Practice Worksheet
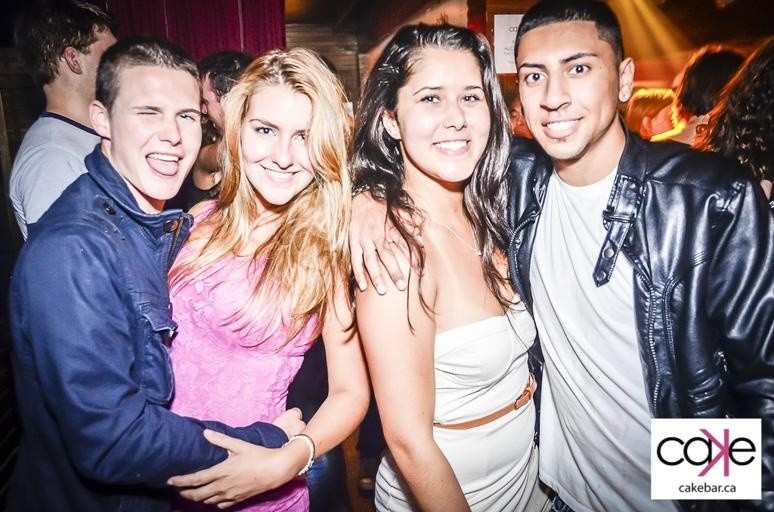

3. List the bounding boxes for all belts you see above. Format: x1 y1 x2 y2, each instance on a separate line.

434 374 537 429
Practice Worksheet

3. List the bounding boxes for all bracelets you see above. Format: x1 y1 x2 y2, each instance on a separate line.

285 433 316 476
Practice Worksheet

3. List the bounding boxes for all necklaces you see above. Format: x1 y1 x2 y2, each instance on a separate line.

438 218 482 259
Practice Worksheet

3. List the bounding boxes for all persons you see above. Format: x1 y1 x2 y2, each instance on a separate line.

160 46 371 510
348 0 774 510
354 20 558 512
9 0 774 238
8 38 308 511
